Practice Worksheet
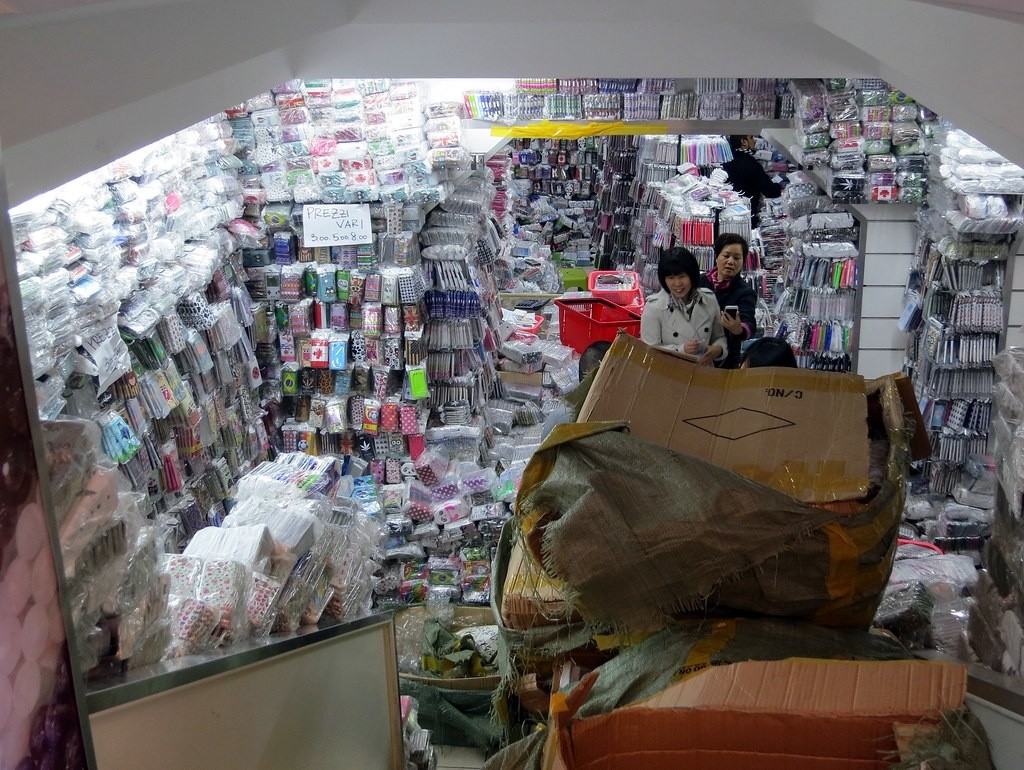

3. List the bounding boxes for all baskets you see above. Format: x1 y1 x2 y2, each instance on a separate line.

500 311 544 341
588 271 645 315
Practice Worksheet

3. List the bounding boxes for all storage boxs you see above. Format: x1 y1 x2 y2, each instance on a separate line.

498 372 543 385
501 332 967 770
395 606 502 690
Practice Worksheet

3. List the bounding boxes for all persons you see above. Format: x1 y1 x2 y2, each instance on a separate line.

723 135 781 230
640 247 728 367
699 233 757 369
540 340 613 444
739 337 798 369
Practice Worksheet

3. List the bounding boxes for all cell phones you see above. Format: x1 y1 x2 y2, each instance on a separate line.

723 306 738 320
